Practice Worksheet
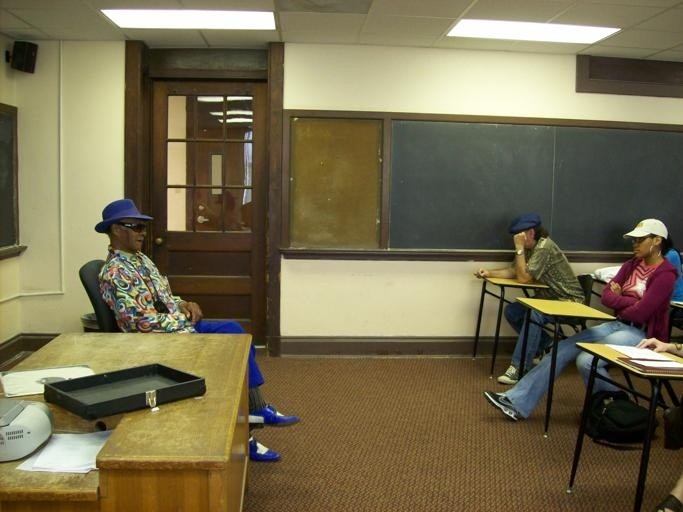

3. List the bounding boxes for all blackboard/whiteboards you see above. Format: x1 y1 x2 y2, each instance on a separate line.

1 104 19 258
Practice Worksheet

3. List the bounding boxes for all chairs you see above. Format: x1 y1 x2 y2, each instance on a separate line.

79 260 120 332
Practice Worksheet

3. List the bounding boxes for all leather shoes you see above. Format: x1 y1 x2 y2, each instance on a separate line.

249 404 299 429
249 436 280 461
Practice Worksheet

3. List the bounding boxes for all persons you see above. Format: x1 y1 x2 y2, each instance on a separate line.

482 217 679 423
634 337 683 358
471 212 586 385
660 234 683 341
650 473 683 512
93 199 302 463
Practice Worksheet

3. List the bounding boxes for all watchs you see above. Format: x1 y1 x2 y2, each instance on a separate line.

513 250 524 256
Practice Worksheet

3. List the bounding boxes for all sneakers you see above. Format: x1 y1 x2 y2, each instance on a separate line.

484 392 519 421
498 342 555 384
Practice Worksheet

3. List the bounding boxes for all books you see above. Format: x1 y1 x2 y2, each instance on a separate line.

615 356 683 375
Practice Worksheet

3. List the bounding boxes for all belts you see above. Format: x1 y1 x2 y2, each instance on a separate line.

618 319 648 332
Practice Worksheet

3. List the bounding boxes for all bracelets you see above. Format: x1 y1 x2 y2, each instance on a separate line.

674 343 682 354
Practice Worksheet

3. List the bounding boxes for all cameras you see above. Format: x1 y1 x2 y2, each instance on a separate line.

153 300 171 314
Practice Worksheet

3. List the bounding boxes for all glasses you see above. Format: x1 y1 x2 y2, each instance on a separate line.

118 222 144 232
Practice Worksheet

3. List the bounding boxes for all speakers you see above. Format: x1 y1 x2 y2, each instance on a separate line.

12 40 39 73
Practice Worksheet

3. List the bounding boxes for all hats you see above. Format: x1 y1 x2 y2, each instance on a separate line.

509 213 540 232
623 218 668 240
95 199 154 232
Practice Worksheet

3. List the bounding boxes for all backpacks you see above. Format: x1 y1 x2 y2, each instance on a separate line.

584 391 658 450
